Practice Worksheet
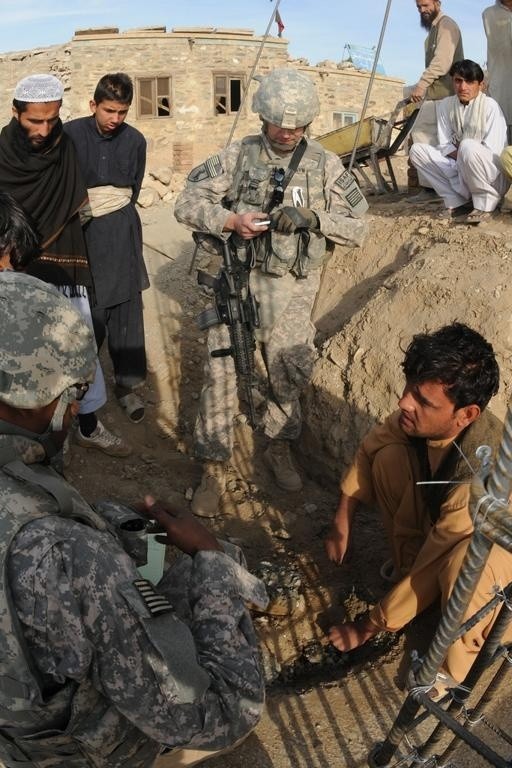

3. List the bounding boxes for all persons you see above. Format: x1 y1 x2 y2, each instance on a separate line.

409 60 510 224
501 146 512 213
172 70 369 519
0 189 44 271
0 73 133 457
63 74 150 424
1 273 271 768
481 0 511 130
408 0 464 107
327 316 512 707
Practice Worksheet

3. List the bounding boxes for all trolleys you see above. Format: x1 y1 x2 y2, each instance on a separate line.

310 95 419 197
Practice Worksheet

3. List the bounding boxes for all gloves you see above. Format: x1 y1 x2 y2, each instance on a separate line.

269 206 318 233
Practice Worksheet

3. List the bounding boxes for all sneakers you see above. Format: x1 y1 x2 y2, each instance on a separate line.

76 420 132 458
404 186 443 204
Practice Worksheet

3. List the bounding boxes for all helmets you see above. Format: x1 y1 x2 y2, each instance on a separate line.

0 271 97 410
251 69 320 130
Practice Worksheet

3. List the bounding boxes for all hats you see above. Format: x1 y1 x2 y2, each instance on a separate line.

14 74 65 103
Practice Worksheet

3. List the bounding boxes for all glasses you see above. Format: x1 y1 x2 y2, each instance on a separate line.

74 382 89 400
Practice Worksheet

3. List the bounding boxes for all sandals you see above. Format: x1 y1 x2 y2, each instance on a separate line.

437 204 472 219
465 207 492 223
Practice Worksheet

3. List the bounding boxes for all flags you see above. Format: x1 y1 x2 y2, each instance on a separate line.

275 9 284 37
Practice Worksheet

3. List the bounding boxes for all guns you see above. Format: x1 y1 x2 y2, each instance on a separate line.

197 241 259 432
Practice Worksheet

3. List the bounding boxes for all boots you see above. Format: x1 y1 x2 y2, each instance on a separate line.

263 440 304 492
190 460 226 518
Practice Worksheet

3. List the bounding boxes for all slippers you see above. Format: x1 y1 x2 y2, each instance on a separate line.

118 392 145 424
